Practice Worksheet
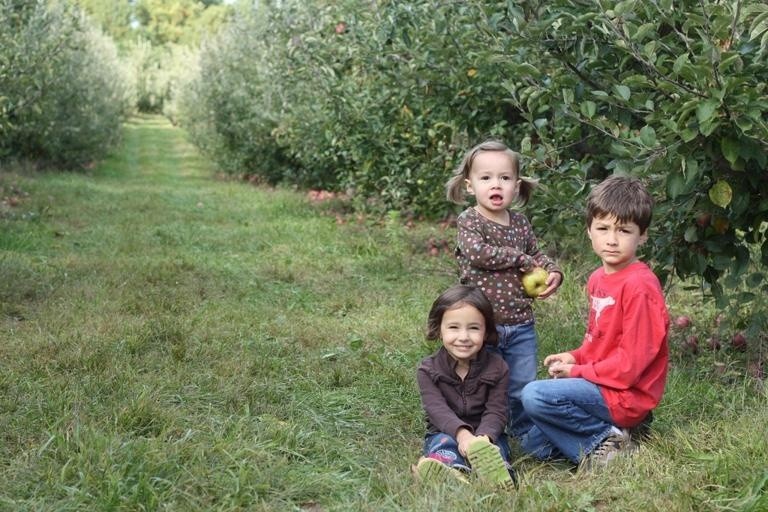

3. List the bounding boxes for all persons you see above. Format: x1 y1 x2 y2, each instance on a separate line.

412 284 520 498
514 174 671 475
441 140 566 440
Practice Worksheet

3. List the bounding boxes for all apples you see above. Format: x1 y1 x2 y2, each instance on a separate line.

714 313 723 327
337 23 346 32
679 334 698 355
218 165 455 256
521 266 550 298
733 334 747 352
676 316 690 327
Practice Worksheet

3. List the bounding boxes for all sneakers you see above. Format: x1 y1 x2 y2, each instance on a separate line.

465 438 516 495
588 427 626 468
414 456 471 495
